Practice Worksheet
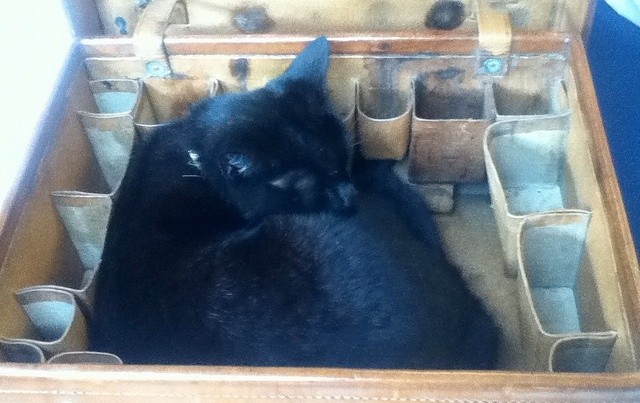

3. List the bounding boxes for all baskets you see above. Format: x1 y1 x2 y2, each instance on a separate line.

0 0 640 403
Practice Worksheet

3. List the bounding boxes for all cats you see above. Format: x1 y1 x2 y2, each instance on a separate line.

86 35 502 369
